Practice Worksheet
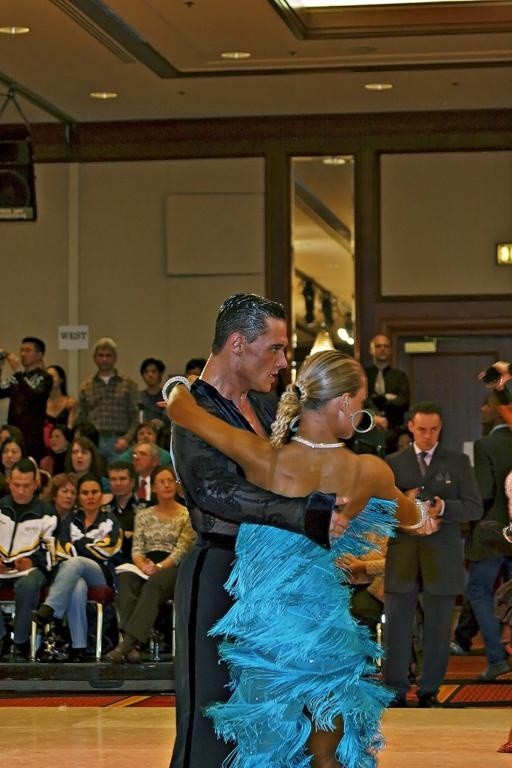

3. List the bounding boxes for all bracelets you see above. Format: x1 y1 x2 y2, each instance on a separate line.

398 497 425 530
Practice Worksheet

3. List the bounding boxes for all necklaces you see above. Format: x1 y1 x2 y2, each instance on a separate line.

291 435 346 449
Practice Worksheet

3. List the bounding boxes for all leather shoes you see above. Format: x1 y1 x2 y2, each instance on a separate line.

417 693 447 708
387 696 407 708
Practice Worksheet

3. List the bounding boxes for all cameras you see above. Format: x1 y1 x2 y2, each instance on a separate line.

482 367 501 384
416 491 435 506
0 351 9 360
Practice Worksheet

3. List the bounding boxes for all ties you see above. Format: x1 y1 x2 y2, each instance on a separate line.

373 371 386 417
138 478 149 500
418 451 430 479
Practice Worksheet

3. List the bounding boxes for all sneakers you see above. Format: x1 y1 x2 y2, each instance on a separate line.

13 642 31 663
60 647 88 663
101 642 128 663
449 641 472 655
130 650 142 661
478 662 511 681
29 604 53 625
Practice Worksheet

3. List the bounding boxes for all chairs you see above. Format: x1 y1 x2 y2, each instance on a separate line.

0 580 175 661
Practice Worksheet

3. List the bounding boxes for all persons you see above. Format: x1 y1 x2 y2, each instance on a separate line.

453 393 510 681
364 335 409 453
377 401 484 708
1 337 208 663
161 351 429 768
478 360 511 418
170 293 349 768
